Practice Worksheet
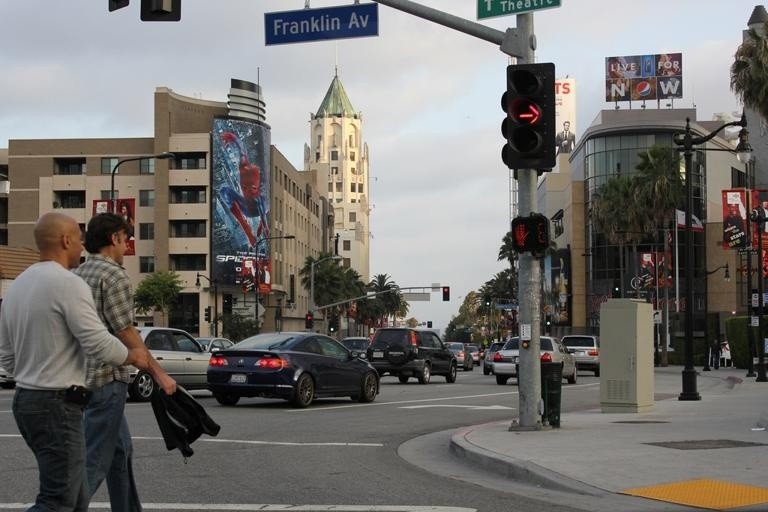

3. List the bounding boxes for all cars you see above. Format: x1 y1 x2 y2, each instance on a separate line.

204 331 380 408
481 334 600 386
127 326 235 403
339 328 480 384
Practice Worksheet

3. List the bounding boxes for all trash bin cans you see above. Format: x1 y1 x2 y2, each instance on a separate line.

515 360 560 427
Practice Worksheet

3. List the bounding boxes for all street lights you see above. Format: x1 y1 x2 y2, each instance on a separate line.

111 152 175 214
255 235 295 334
310 256 342 332
195 272 218 337
673 109 753 401
703 262 730 371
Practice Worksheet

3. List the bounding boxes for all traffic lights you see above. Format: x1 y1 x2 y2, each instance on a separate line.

443 287 450 301
305 313 314 329
328 321 337 333
499 63 556 168
510 213 551 255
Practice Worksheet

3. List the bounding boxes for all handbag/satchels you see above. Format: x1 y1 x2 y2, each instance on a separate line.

65 385 93 411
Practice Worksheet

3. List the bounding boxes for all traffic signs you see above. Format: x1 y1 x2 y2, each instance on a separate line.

476 0 562 21
264 3 380 46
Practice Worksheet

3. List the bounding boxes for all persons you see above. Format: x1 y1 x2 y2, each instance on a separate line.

118 203 135 227
0 212 152 512
67 213 177 512
754 192 768 235
